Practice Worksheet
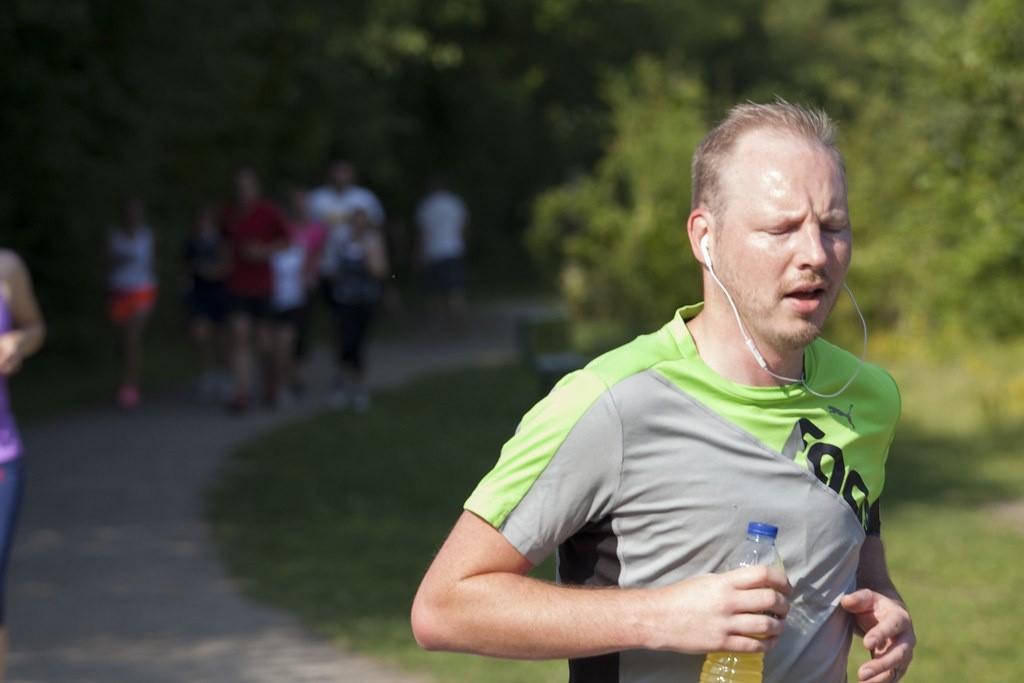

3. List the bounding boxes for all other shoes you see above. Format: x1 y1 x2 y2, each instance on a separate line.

118 386 141 408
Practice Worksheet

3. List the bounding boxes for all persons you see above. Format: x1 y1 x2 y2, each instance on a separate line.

410 101 917 683
204 155 390 414
414 173 474 327
0 248 45 683
107 196 158 412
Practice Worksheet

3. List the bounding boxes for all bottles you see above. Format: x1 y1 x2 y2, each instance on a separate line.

699 523 786 683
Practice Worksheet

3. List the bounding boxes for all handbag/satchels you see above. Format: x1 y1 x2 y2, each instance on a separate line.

333 268 378 303
